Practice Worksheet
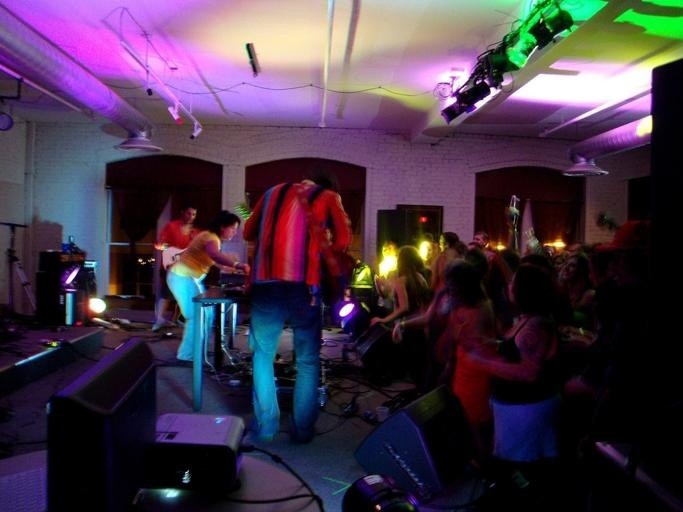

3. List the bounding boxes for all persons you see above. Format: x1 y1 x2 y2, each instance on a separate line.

371 210 646 468
149 205 204 331
165 209 251 364
242 173 353 443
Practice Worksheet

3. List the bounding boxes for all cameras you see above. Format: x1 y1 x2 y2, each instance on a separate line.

342 474 418 511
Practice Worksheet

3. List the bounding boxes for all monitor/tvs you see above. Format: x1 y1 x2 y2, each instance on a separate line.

45 337 154 511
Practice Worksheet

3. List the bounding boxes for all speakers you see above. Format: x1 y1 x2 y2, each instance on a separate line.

353 322 407 371
376 209 405 243
355 382 475 505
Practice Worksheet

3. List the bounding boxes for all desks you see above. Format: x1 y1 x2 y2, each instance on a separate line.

0 449 323 512
185 291 330 415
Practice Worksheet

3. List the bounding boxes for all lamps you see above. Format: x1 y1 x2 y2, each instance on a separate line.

111 129 163 154
120 40 206 141
87 292 108 329
563 154 610 180
334 297 361 323
439 0 573 127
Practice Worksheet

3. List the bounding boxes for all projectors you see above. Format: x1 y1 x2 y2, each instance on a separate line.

151 412 246 494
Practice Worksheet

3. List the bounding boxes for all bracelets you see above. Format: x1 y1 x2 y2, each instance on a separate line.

231 261 240 270
